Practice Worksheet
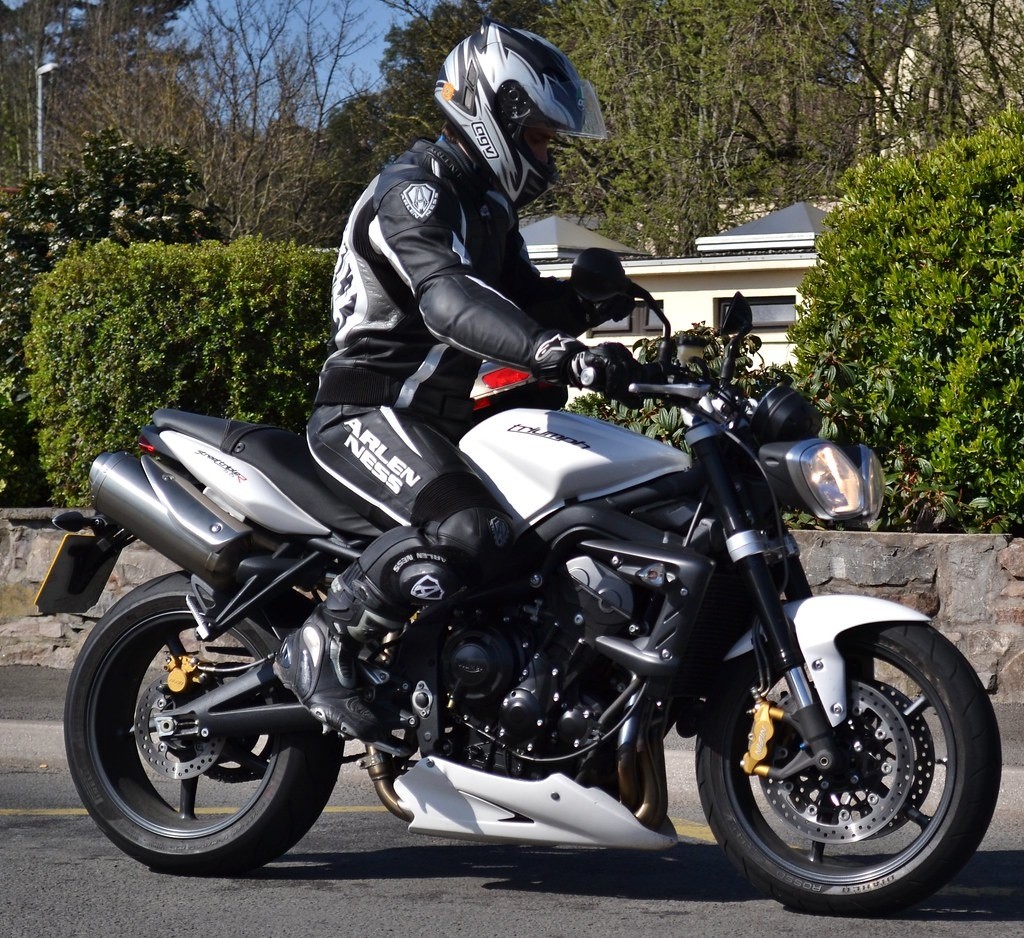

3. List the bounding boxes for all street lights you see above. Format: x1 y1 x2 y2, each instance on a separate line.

35 61 60 174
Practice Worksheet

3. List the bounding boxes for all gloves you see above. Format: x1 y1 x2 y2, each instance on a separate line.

568 346 647 408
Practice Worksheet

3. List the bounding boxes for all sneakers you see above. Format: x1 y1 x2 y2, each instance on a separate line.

274 611 378 745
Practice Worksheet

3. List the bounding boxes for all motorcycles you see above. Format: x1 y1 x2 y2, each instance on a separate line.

31 243 1003 912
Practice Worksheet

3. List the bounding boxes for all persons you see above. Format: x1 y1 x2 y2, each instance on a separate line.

270 23 658 744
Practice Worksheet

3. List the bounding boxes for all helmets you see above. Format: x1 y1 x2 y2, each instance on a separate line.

433 17 607 210
